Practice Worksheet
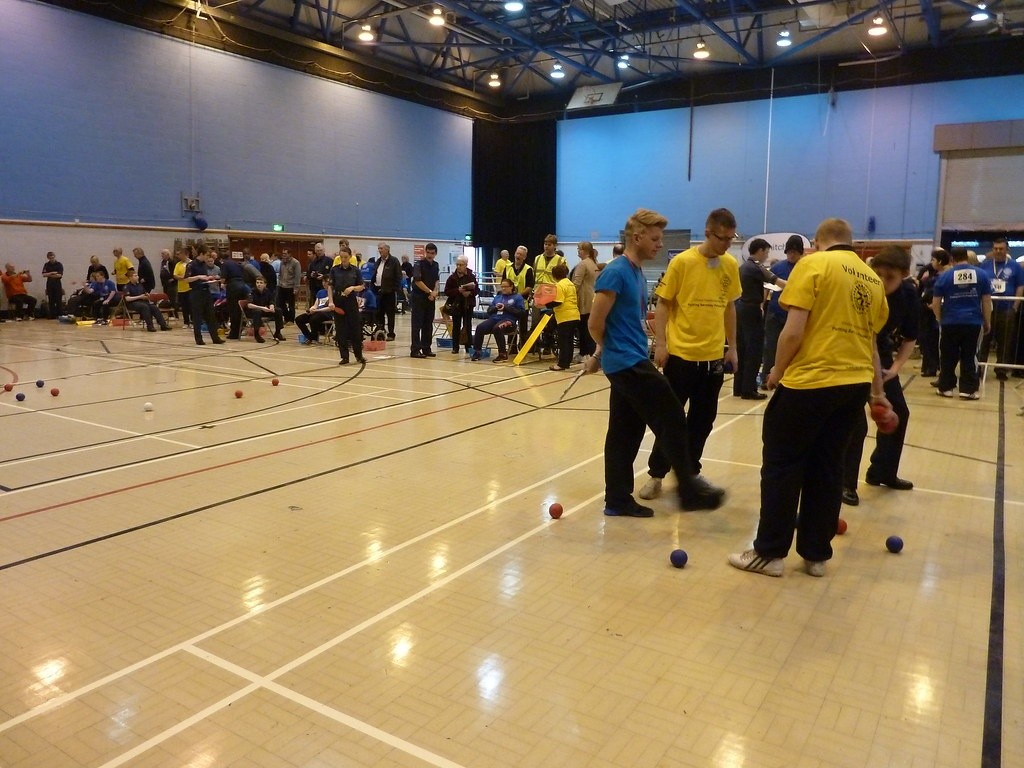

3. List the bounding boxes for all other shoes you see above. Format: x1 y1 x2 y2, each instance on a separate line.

411 352 425 358
690 474 724 496
930 379 940 387
452 350 459 354
492 353 509 363
424 352 436 357
549 365 564 370
15 313 395 365
734 391 767 400
472 353 480 362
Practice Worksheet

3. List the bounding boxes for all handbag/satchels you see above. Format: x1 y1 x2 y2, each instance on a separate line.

443 298 458 316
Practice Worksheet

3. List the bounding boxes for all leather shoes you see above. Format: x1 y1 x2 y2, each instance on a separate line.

864 472 913 490
842 486 859 506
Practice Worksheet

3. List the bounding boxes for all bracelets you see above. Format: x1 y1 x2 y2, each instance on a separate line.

592 353 600 361
428 289 432 294
872 393 887 399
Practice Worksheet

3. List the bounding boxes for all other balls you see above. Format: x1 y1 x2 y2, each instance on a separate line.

670 549 688 568
36 380 44 388
51 388 60 396
272 379 279 385
549 503 563 518
16 393 25 401
837 519 848 535
144 402 153 410
886 536 904 553
877 410 898 434
235 390 243 397
4 383 13 392
871 406 885 421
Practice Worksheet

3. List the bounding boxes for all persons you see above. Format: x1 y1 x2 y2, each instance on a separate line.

240 246 301 343
733 233 804 399
917 238 1024 399
444 255 479 354
58 256 122 326
841 243 920 506
588 208 725 518
355 252 376 322
42 252 64 319
111 247 177 332
728 219 890 576
398 254 413 315
329 239 366 365
303 244 334 302
409 242 440 358
168 244 245 345
295 274 334 345
471 234 607 371
638 207 743 501
370 242 402 341
2 261 38 321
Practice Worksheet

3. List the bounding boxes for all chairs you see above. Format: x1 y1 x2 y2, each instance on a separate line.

482 319 523 360
238 300 277 340
432 313 453 346
306 315 337 347
644 311 656 370
6 288 178 331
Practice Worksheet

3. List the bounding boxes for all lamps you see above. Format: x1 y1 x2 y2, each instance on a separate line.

971 0 989 22
776 24 791 46
693 37 709 59
617 49 631 68
550 60 565 78
358 19 374 41
867 11 888 36
429 4 445 26
488 70 501 87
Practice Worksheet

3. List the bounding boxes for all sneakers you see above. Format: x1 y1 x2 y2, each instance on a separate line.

682 490 721 511
728 550 784 577
604 494 654 518
959 392 979 399
638 477 662 499
803 561 826 577
936 390 953 397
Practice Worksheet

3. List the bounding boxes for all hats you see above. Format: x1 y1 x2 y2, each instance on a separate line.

784 235 804 256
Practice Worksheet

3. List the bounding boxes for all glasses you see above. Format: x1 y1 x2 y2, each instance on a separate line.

501 286 510 288
711 232 739 244
456 263 465 266
